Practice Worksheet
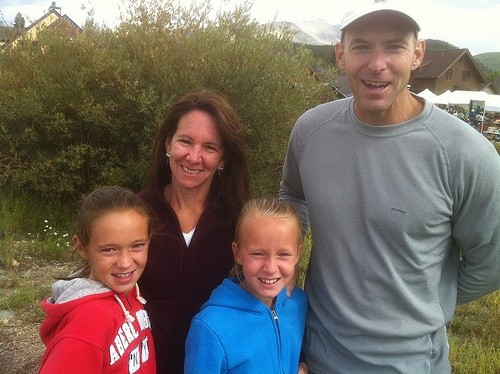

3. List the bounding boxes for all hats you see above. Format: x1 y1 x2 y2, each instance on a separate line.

339 0 420 30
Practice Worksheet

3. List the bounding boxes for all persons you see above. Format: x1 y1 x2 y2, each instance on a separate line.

37 186 160 373
184 196 309 373
134 91 310 374
278 1 500 373
